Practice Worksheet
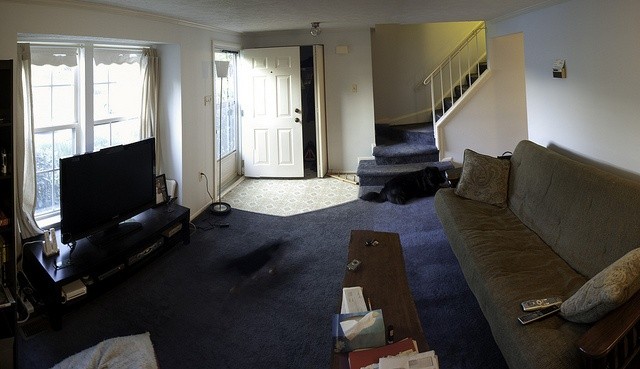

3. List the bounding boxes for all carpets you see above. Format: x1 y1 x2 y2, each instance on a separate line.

13 190 508 369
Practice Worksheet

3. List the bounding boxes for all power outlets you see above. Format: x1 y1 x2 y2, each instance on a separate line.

200 170 205 176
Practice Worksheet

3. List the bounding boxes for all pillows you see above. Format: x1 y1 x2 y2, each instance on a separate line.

560 247 640 324
453 149 510 208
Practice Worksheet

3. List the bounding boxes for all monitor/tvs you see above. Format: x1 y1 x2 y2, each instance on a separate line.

521 295 564 311
60 136 157 244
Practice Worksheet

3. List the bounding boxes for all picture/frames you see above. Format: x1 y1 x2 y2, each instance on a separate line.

156 173 169 209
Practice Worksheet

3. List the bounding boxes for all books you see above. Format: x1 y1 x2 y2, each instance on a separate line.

348 336 439 369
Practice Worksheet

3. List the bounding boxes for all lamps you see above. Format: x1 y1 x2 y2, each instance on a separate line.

310 22 321 37
208 61 231 217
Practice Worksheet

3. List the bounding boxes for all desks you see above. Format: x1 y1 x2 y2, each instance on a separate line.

22 200 190 332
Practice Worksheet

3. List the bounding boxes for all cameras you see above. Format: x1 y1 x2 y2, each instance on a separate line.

346 259 363 271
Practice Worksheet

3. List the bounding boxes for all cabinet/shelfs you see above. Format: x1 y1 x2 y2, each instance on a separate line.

0 60 12 369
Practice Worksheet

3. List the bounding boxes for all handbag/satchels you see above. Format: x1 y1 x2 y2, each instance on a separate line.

497 151 513 160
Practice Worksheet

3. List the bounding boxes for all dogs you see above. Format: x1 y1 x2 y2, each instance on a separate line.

358 165 446 206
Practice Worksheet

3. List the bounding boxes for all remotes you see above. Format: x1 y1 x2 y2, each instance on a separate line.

519 305 569 324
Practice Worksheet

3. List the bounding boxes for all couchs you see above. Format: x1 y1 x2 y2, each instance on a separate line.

435 140 639 369
